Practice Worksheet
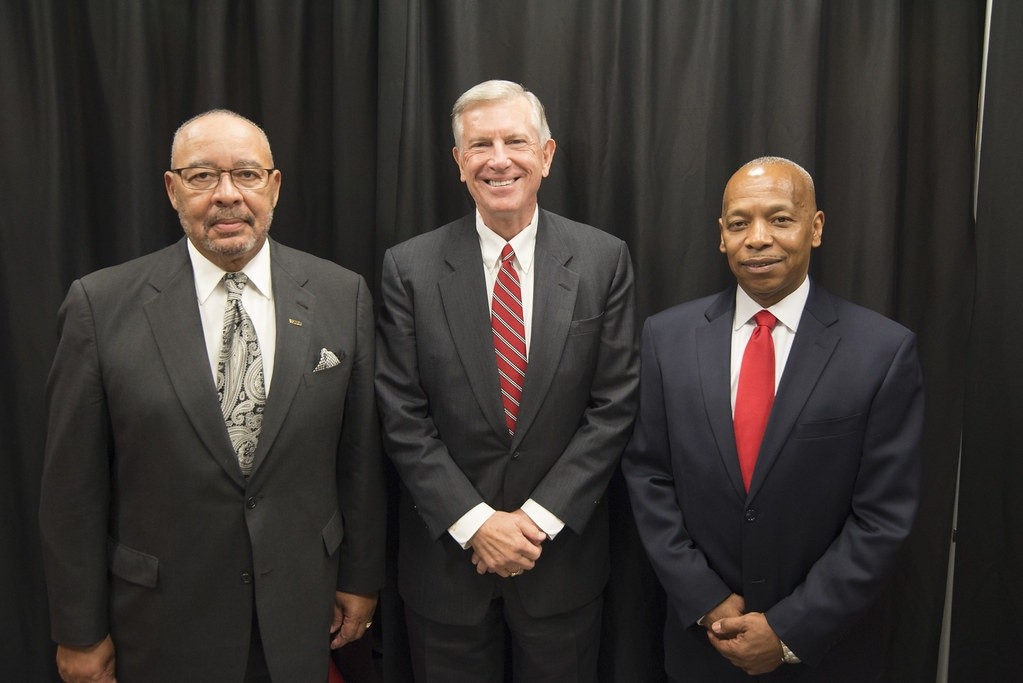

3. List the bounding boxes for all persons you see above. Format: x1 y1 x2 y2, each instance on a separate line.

40 110 387 683
372 81 639 683
621 155 923 683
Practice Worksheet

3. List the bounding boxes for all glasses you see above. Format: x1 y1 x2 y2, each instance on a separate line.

171 167 276 191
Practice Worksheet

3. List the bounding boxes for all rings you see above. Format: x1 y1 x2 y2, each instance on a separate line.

366 622 372 628
511 567 520 576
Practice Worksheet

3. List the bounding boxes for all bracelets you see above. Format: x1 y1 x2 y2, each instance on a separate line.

782 642 800 664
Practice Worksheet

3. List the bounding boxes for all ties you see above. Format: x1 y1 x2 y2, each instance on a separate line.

216 271 266 478
734 310 777 496
490 245 528 439
